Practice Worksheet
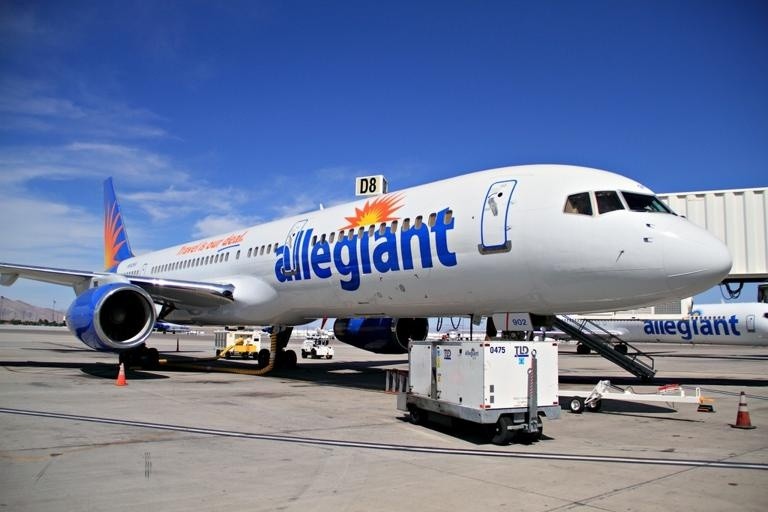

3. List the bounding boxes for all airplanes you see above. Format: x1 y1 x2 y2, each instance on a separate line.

0 161 734 386
532 296 768 355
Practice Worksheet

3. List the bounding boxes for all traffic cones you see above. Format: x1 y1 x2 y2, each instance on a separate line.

730 388 755 429
115 361 127 386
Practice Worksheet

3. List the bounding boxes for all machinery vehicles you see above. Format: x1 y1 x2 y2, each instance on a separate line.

301 328 337 362
